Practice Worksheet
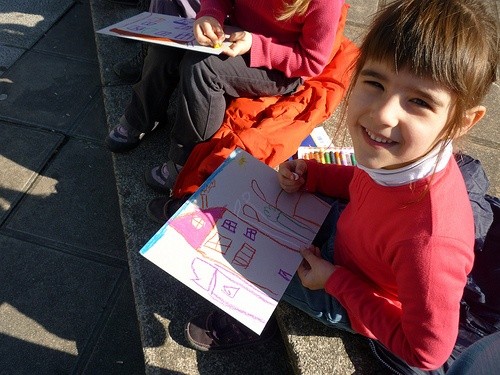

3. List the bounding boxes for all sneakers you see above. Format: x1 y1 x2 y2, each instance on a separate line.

144 162 172 193
104 114 148 153
112 42 148 82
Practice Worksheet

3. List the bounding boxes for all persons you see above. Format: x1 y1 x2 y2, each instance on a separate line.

145 0 499 370
104 0 345 194
112 0 200 83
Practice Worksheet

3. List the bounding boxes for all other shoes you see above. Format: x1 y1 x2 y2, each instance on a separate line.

184 312 276 351
146 193 190 224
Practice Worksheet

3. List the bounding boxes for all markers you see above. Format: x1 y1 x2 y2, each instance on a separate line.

351 153 356 166
305 151 346 165
